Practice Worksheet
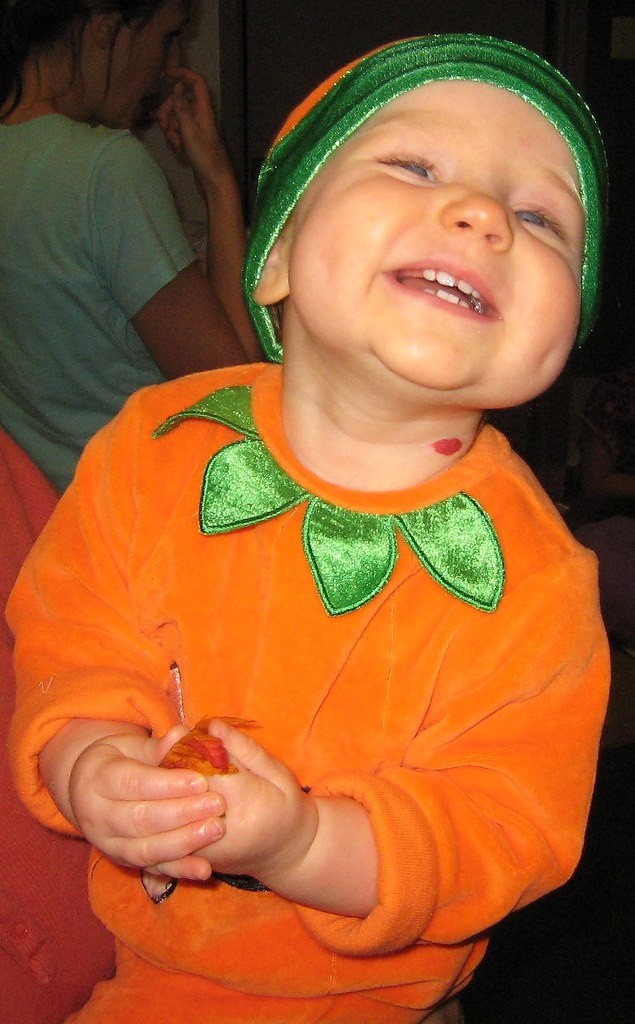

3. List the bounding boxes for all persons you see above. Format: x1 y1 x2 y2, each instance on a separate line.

0 427 461 1024
5 33 610 1024
0 0 270 492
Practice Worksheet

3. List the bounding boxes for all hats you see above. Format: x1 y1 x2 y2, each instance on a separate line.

240 32 611 364
